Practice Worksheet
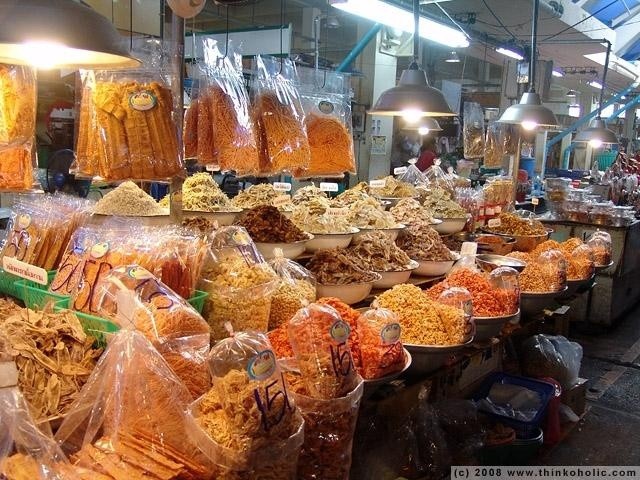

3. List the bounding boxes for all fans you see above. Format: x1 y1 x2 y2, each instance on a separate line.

46 149 90 198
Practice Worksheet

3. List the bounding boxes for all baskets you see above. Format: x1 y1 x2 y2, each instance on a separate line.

0 267 209 351
556 169 586 180
466 371 555 439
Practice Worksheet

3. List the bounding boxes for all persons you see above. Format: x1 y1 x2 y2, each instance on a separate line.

415 136 437 172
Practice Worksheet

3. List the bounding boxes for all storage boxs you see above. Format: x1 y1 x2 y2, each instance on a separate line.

561 379 588 416
428 343 505 400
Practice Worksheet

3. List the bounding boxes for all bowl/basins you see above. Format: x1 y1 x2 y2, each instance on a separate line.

81 191 614 392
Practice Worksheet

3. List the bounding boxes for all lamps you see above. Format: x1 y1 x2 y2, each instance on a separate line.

1 0 143 70
